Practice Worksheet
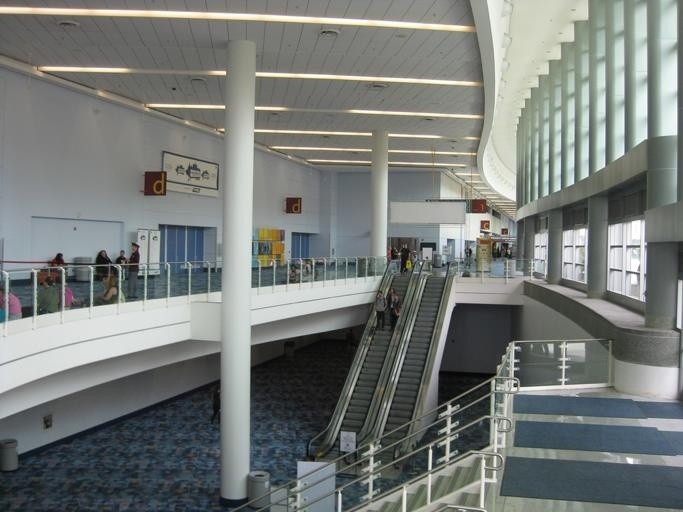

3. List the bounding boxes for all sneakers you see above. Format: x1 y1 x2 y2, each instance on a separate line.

376 326 395 331
126 295 137 300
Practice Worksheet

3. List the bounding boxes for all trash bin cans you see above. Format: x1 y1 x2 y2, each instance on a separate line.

434 253 443 268
73 257 93 282
0 439 19 471
248 471 271 508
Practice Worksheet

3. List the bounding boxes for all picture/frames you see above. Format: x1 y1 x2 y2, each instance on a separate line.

162 151 219 190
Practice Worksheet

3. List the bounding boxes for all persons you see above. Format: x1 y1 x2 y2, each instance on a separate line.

37 254 73 313
96 242 140 304
493 246 513 261
392 293 401 327
387 288 398 313
0 286 22 322
211 388 221 423
464 246 472 268
388 244 410 272
376 291 387 329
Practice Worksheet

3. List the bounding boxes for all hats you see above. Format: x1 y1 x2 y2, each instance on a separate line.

132 242 140 248
